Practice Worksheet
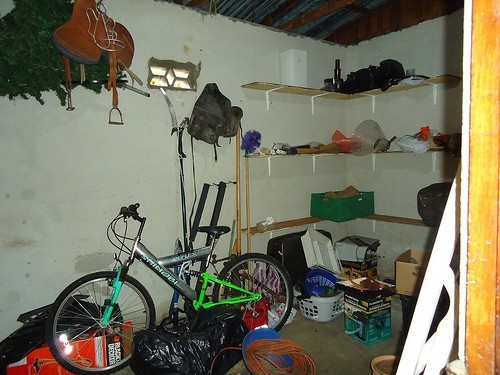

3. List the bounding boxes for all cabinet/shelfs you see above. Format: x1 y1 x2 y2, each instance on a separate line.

240 73 462 303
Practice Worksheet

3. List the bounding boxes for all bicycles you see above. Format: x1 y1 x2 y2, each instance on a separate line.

41 202 295 375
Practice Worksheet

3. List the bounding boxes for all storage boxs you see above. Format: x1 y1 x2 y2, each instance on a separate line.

6 320 134 375
394 248 432 298
309 191 374 224
336 278 394 346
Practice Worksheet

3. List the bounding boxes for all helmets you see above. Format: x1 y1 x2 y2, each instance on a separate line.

242 328 293 375
305 268 340 296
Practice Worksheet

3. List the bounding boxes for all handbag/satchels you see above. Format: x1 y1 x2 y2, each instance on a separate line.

132 307 250 375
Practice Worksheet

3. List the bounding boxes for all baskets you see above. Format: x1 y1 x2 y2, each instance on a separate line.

292 282 344 322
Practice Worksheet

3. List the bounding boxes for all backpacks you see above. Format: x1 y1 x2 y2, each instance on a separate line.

187 83 243 144
341 59 407 94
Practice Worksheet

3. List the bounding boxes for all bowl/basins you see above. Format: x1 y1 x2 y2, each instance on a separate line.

240 328 294 375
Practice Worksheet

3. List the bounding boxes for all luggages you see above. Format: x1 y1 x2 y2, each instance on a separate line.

266 229 333 297
